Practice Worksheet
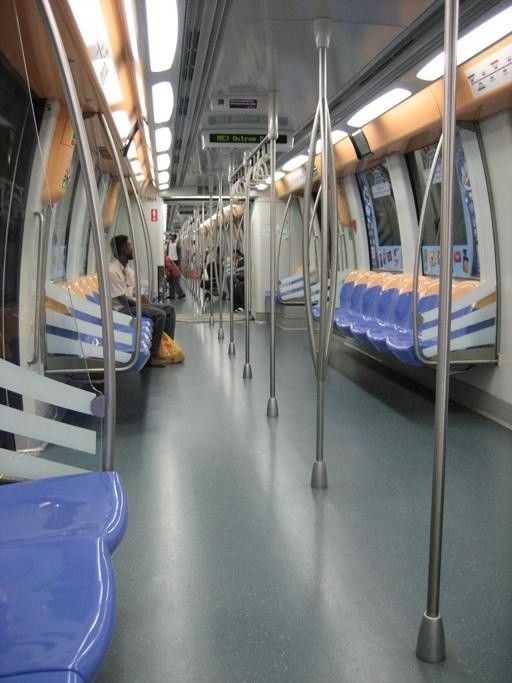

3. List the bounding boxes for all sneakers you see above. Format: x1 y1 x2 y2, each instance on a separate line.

149 357 165 364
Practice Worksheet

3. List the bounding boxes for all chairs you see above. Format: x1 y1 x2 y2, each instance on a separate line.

0 536 117 682
0 669 88 682
266 265 315 305
312 270 480 367
44 271 154 373
0 470 128 553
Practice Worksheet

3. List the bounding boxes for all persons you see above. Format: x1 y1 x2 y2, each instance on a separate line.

162 251 186 299
167 232 181 265
200 243 246 312
106 235 177 368
